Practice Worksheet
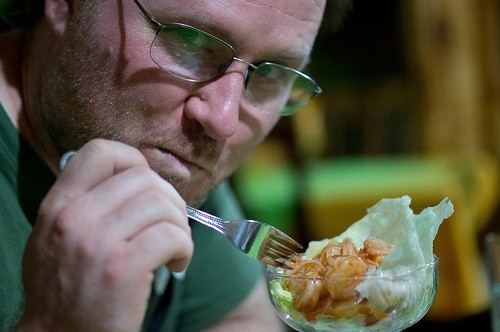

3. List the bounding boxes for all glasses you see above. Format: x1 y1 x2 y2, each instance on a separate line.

134 0 322 116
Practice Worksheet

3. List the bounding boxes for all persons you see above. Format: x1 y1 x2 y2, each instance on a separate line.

0 1 331 332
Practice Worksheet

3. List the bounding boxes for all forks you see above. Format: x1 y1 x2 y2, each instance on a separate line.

60 151 303 271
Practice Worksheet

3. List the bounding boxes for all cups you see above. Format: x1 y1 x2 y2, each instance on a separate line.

266 248 439 332
